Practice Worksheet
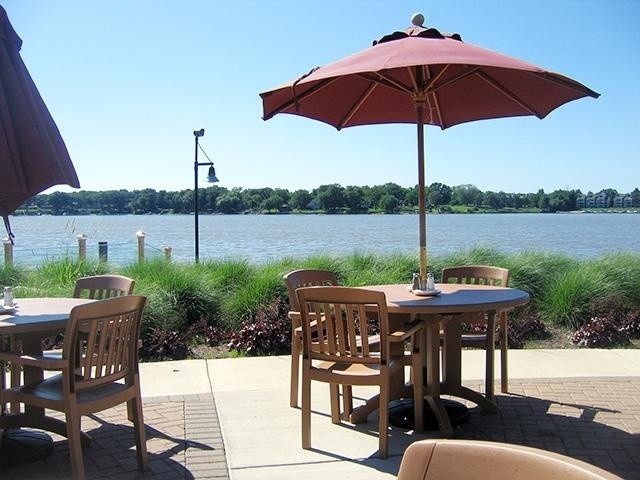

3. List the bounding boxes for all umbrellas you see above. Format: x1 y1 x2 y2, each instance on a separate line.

260 13 602 385
0 7 82 216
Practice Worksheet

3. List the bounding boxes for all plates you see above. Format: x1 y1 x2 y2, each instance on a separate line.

412 290 441 295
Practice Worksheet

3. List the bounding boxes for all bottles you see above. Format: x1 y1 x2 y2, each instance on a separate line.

3 286 14 307
427 272 435 290
412 273 419 290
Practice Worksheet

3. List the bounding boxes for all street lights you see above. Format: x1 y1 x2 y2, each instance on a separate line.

194 129 219 262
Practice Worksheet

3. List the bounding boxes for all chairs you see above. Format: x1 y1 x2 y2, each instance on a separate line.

284 270 356 421
397 439 621 480
295 286 424 459
73 273 145 422
443 264 511 400
10 294 151 480
